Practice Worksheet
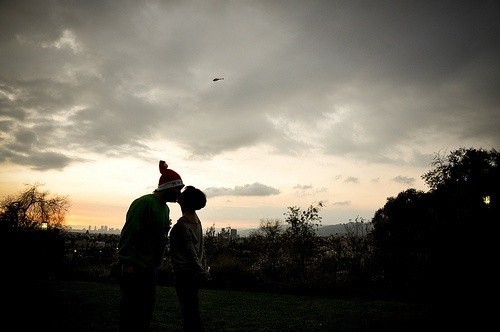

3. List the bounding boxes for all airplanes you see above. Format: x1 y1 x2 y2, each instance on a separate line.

213 78 224 81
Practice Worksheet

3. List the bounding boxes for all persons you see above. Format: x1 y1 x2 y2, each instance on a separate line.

111 161 185 332
167 186 213 332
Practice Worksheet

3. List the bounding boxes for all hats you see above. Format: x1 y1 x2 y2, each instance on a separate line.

158 160 185 191
